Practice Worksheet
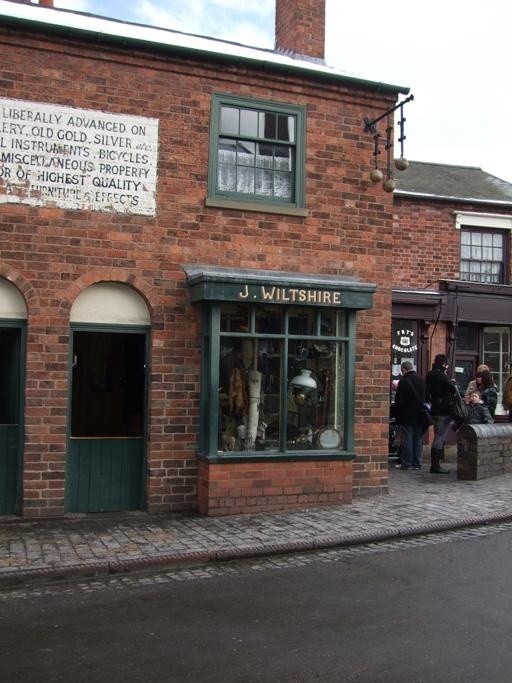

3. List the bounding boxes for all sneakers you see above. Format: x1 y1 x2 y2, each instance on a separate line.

394 462 424 470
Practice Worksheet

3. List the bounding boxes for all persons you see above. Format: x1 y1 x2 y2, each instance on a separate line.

392 354 498 475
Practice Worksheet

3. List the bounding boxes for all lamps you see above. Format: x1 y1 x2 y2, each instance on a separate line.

364 93 414 192
290 369 318 389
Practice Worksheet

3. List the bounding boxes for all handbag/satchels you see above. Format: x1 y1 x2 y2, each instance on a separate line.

421 402 433 425
451 395 468 420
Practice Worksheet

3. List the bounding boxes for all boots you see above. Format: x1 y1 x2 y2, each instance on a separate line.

430 446 450 474
